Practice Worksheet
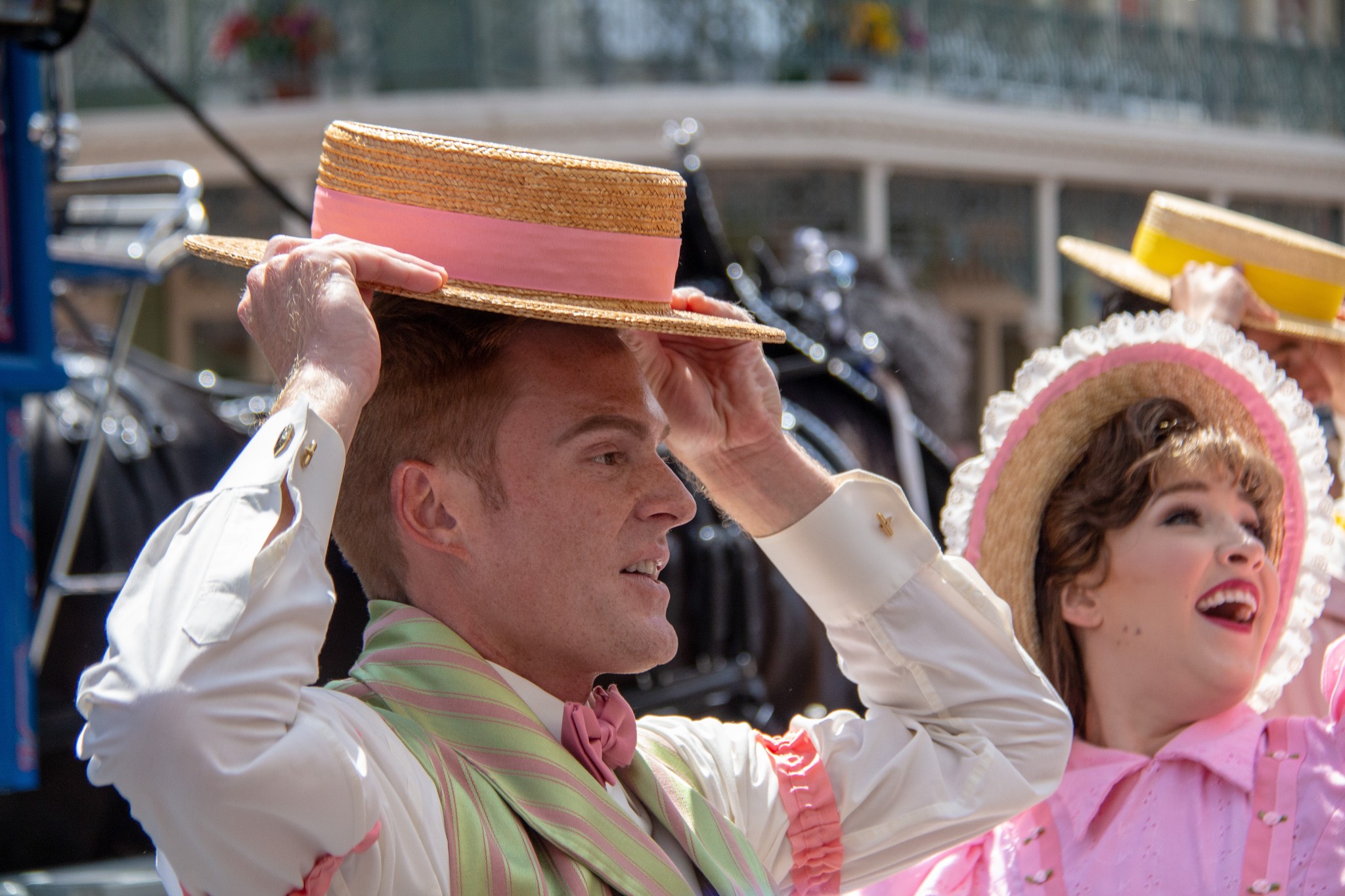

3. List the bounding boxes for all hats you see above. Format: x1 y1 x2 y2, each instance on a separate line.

183 121 788 347
1057 188 1345 348
941 310 1338 714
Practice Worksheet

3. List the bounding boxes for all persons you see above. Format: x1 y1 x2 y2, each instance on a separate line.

860 309 1345 896
74 123 1074 896
1056 189 1345 723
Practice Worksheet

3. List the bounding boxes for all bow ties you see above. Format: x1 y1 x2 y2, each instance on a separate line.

563 684 637 794
